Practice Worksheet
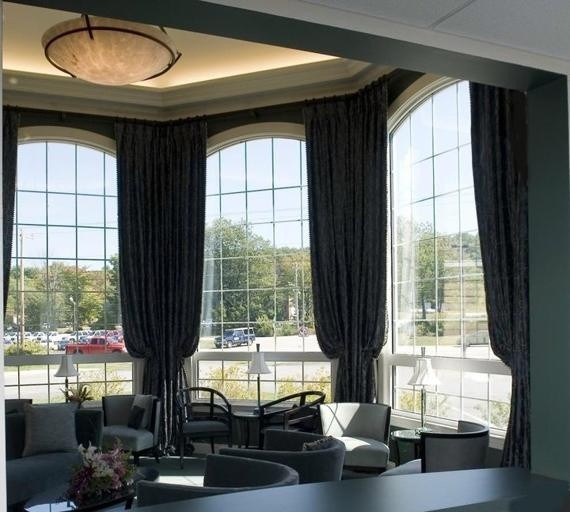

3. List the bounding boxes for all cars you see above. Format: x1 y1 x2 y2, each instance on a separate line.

455 329 489 347
43 322 51 329
4 329 123 350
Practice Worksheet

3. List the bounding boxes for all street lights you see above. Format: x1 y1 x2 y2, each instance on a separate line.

69 295 77 332
451 244 470 265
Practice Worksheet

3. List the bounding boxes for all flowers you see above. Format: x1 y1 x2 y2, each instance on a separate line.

67 437 134 501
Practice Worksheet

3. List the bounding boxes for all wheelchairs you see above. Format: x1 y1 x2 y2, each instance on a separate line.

297 328 310 337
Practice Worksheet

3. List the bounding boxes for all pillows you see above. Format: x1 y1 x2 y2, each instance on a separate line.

130 394 153 430
302 435 333 451
22 401 80 456
127 406 145 430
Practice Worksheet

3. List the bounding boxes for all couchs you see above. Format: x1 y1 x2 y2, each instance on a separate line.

5 409 104 505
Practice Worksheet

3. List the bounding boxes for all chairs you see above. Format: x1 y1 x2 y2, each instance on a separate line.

219 428 346 483
258 391 325 448
101 395 161 466
378 420 489 476
175 387 232 456
318 402 391 473
136 454 298 508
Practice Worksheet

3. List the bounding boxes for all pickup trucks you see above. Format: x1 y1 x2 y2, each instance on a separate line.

64 335 125 355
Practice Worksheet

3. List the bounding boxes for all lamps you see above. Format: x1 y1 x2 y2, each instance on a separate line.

245 343 271 414
41 13 180 87
53 346 80 403
407 347 438 434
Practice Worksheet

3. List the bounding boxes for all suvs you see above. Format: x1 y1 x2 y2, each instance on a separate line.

214 326 257 349
